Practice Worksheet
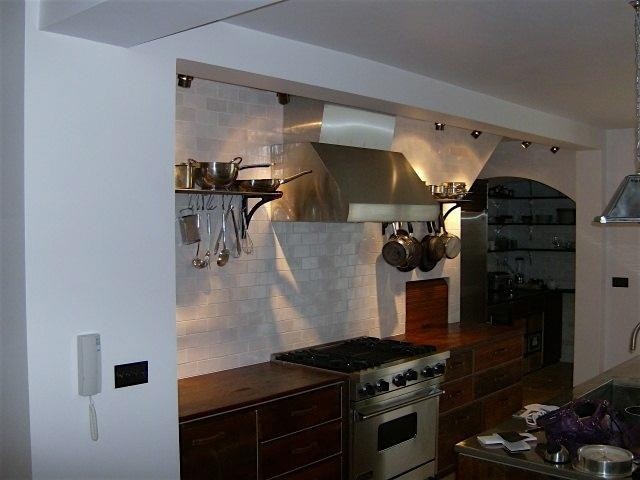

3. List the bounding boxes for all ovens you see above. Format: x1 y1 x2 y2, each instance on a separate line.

353 396 439 480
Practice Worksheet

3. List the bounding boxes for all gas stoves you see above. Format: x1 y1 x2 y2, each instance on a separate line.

275 336 451 408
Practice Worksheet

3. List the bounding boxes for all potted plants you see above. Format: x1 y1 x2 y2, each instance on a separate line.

495 235 509 250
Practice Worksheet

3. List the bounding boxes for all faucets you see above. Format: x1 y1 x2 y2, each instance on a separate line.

630 322 640 351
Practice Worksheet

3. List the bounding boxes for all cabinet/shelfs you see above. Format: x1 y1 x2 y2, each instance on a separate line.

178 361 351 480
382 320 524 479
488 195 576 253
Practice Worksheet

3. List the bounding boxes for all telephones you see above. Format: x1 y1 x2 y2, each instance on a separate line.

522 403 561 420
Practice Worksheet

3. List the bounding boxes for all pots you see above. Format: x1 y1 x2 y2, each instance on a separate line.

175 157 312 192
382 182 473 272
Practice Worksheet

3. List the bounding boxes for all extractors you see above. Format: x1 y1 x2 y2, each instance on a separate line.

271 142 441 224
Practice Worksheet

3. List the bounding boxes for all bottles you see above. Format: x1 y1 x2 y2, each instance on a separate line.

584 399 608 430
179 207 200 245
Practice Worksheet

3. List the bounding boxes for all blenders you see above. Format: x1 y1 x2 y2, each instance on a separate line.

515 258 525 289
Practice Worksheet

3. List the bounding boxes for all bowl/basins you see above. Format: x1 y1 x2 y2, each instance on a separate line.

573 446 634 474
557 208 575 224
623 406 640 425
520 215 552 224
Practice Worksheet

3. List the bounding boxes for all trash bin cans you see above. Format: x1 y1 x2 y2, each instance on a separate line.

488 271 513 313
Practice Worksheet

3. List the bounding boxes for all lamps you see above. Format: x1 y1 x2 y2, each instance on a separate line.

278 93 289 105
435 123 444 130
471 130 481 139
178 74 193 88
521 142 531 149
550 146 560 154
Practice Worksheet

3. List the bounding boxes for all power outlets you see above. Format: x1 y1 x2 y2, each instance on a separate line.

114 360 148 388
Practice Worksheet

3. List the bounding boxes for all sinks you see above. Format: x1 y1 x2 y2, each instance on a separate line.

579 379 639 416
